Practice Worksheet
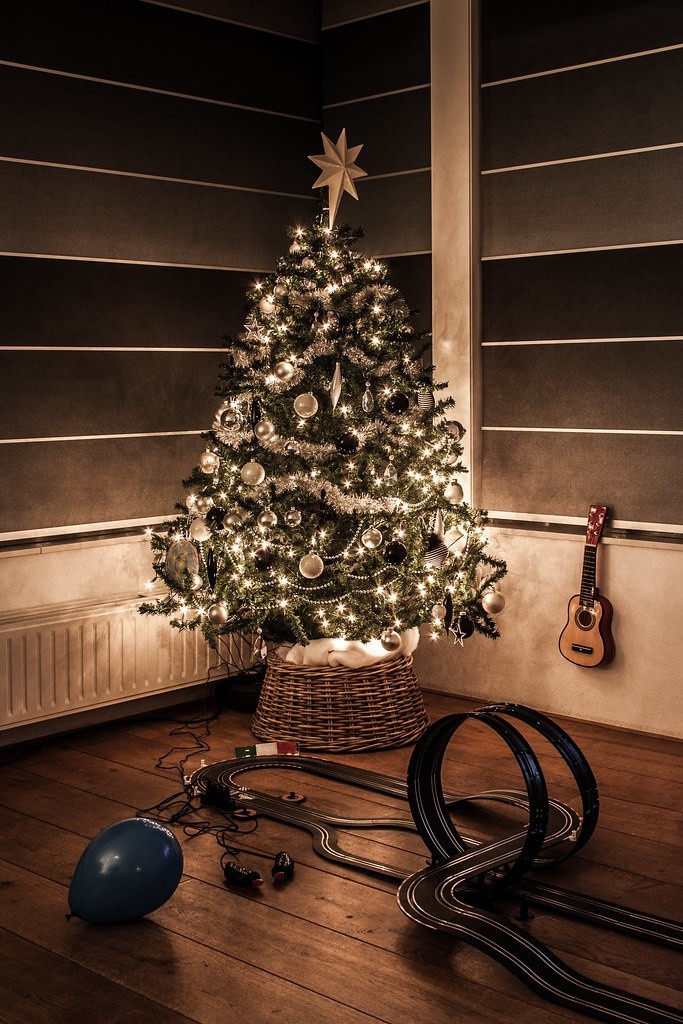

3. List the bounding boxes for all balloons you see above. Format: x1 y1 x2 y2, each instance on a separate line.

64 818 185 925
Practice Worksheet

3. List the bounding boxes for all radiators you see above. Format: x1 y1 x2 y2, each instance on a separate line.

0 584 267 736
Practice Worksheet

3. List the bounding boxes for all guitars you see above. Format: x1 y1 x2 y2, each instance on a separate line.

559 504 616 668
